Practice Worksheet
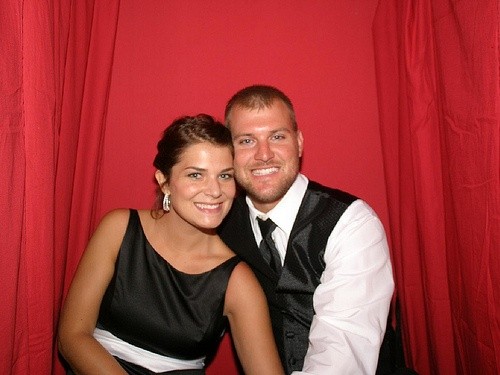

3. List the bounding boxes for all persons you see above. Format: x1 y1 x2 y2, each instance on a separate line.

58 114 286 375
217 85 416 375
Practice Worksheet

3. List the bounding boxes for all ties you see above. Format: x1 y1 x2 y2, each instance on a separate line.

257 217 284 272
